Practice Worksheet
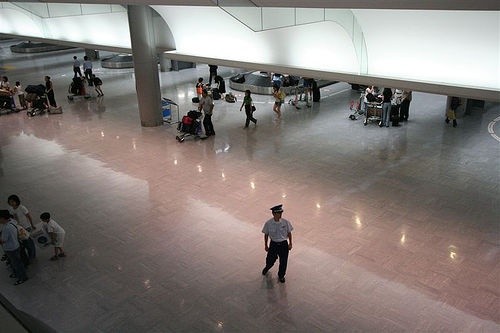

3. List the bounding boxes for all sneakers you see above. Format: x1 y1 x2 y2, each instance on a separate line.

58 252 65 257
50 255 58 261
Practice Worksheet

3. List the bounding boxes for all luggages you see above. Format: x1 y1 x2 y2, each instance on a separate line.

389 103 399 121
211 87 220 100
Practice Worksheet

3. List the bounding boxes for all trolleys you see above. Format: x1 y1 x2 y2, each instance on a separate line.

67 77 91 101
0 100 19 112
176 107 203 142
349 94 363 120
27 92 49 117
364 102 384 127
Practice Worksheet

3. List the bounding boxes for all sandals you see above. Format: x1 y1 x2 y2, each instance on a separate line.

13 280 25 285
9 273 17 278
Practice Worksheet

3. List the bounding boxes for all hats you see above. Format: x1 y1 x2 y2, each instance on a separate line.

269 204 283 213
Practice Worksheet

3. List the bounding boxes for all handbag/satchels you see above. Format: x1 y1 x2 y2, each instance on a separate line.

67 76 83 95
49 105 62 113
10 222 30 241
251 103 256 111
24 83 49 108
280 99 284 102
225 92 237 102
181 110 202 135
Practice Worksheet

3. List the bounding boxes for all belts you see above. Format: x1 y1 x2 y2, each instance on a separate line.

275 243 282 245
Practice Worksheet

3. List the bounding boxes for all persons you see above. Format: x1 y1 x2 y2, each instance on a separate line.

262 204 293 283
196 78 204 113
199 89 215 136
214 76 226 100
270 72 320 118
14 81 28 110
7 195 36 233
45 76 57 107
39 212 65 261
363 85 412 128
2 76 10 90
239 90 257 128
444 96 460 128
73 55 82 77
83 56 93 86
208 64 218 86
0 209 28 285
91 74 104 97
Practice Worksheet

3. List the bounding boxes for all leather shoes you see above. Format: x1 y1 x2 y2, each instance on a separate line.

263 267 268 275
277 273 286 283
